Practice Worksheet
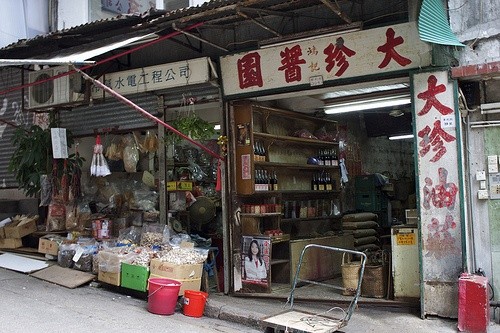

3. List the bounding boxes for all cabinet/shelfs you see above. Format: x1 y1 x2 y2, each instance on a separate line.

234 104 341 195
239 194 354 288
174 163 217 184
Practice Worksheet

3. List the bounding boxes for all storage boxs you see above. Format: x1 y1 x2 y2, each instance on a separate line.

167 181 192 211
355 175 387 211
0 218 203 295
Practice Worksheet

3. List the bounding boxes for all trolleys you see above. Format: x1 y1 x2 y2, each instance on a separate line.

260 244 367 333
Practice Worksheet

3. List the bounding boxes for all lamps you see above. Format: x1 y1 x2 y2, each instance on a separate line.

481 102 500 114
389 110 404 117
322 94 411 114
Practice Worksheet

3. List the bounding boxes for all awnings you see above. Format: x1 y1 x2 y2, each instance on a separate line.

0 27 171 66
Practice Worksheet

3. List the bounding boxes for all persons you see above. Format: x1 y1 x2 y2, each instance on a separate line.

244 240 267 282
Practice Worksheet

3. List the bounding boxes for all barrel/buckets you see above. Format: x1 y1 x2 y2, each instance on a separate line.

182 290 208 318
147 278 181 315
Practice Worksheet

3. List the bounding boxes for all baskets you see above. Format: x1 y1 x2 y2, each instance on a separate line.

340 252 364 295
361 249 388 298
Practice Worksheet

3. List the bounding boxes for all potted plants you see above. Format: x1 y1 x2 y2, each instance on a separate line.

164 114 217 144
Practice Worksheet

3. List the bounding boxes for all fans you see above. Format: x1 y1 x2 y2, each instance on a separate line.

189 196 216 231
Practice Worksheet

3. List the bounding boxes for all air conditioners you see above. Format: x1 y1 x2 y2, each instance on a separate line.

29 65 81 107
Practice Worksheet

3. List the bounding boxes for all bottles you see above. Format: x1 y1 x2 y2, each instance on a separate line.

311 172 332 191
284 200 333 218
254 170 278 191
254 141 265 162
318 148 339 166
